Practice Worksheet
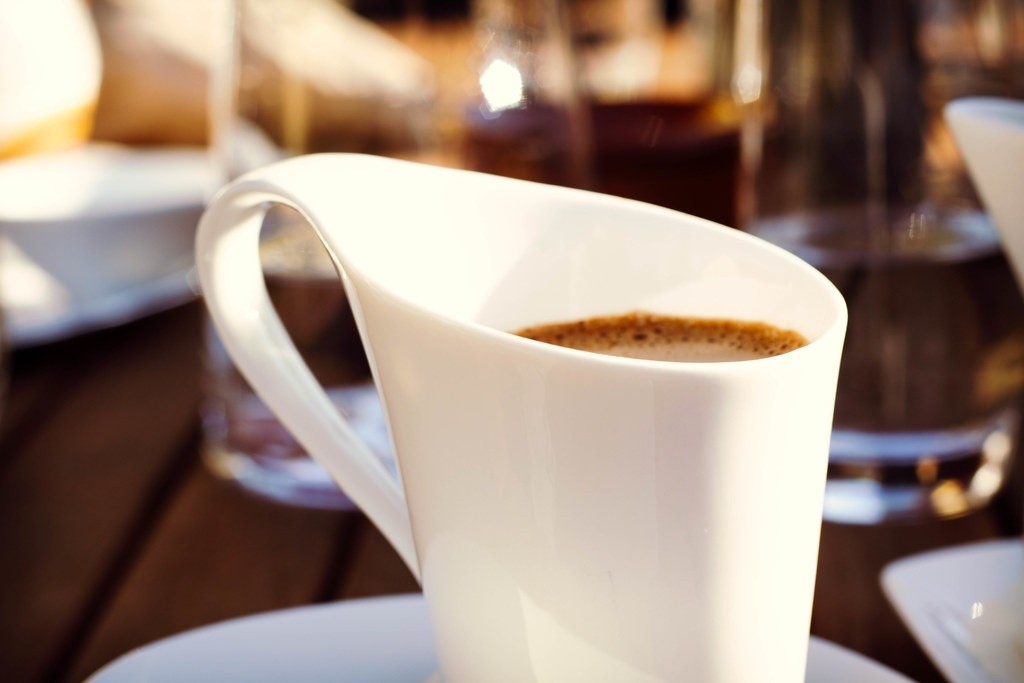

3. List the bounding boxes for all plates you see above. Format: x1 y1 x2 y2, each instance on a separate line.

86 591 909 683
873 545 1024 683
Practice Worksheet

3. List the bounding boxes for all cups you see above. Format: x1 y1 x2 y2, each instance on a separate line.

942 97 1024 283
200 0 590 513
734 92 1009 523
192 156 847 683
